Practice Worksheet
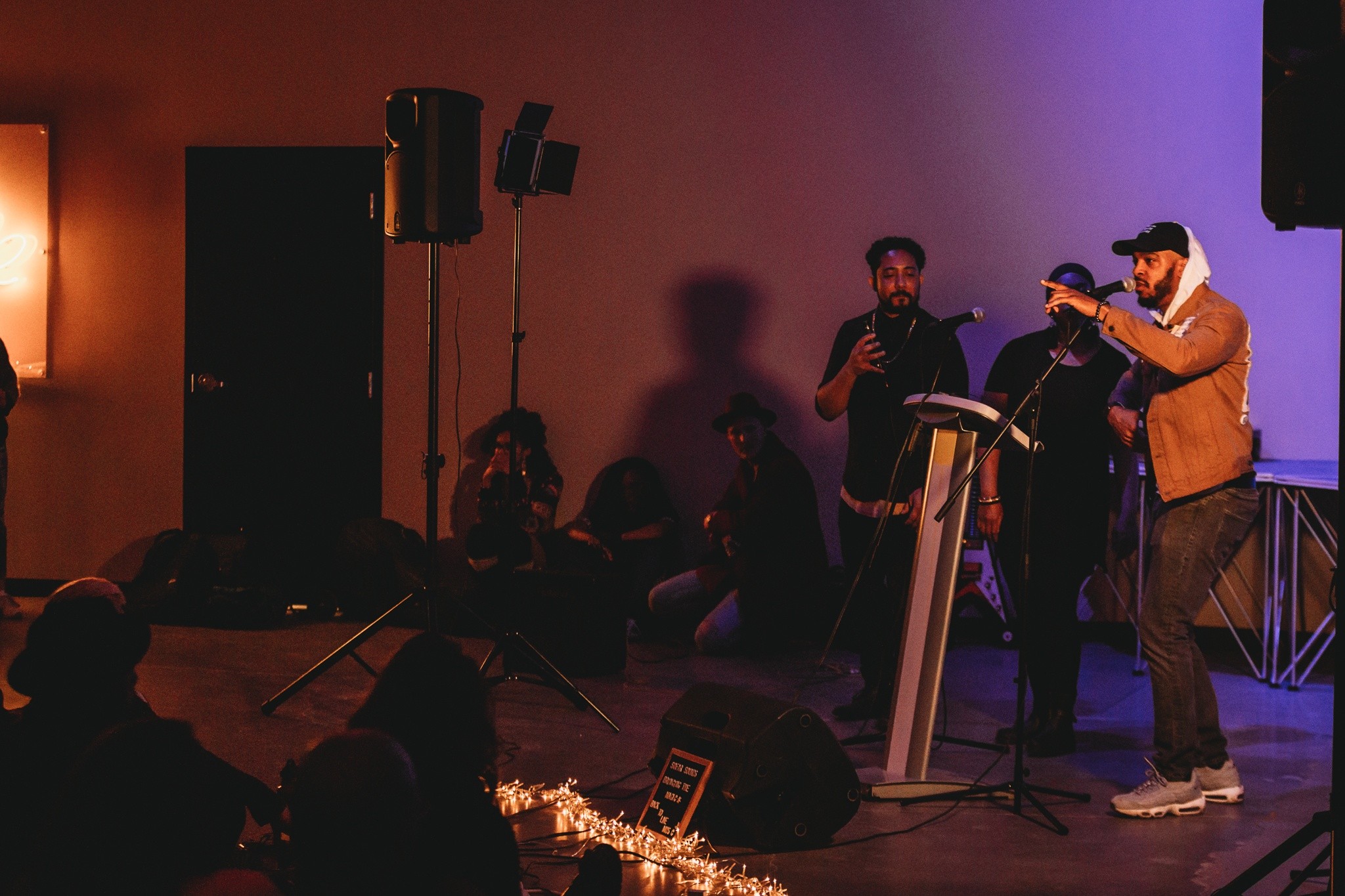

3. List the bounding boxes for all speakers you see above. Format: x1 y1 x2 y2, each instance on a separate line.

125 526 282 629
649 679 862 851
385 88 484 245
1263 0 1345 231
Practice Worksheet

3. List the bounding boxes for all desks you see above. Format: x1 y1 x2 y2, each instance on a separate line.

1081 460 1337 688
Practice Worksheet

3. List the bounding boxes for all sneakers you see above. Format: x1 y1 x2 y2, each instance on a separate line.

1024 708 1075 757
1191 758 1244 803
997 709 1048 744
1111 757 1206 818
832 684 893 722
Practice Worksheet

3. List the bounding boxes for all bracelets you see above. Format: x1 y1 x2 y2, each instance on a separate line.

1095 301 1110 322
1104 403 1120 417
978 495 1001 505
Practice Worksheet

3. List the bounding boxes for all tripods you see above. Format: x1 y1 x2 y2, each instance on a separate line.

836 316 1093 834
261 244 623 738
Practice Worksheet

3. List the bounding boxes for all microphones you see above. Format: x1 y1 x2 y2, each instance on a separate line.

937 307 985 327
1086 276 1138 299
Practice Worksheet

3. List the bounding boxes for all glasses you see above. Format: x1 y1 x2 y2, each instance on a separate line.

495 442 511 450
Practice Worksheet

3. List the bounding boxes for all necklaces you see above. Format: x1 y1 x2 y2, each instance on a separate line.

872 313 916 363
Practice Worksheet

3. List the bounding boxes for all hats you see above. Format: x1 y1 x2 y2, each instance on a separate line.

712 393 779 433
6 577 151 698
1112 221 1190 258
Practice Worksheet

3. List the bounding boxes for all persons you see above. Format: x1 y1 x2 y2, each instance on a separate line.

281 729 622 896
562 458 679 641
0 596 278 896
815 236 970 721
1039 222 1260 818
976 263 1132 753
0 339 19 619
476 430 564 535
647 393 828 650
